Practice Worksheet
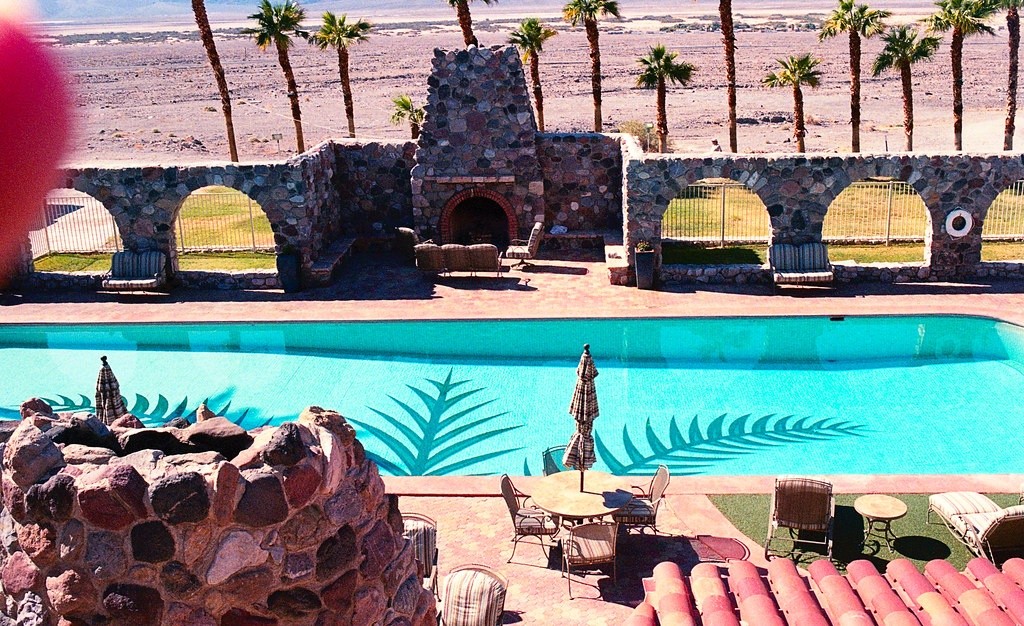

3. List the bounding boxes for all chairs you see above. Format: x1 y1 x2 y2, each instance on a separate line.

505 222 542 269
401 512 441 603
435 563 509 626
499 445 670 602
925 490 1024 568
395 226 433 269
763 477 837 565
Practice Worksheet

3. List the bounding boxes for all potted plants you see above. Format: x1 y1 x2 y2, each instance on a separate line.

634 240 654 289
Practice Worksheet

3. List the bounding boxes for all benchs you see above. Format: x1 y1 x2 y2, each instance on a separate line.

544 227 627 287
768 243 835 293
412 241 503 284
310 234 357 285
353 232 399 250
99 251 169 291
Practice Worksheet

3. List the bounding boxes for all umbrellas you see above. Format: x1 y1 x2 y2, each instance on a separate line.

562 343 599 492
93 354 130 423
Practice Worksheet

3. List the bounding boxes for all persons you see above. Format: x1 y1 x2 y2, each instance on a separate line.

711 139 723 152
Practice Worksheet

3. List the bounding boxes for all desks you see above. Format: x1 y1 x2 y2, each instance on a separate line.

530 469 634 528
853 494 908 556
